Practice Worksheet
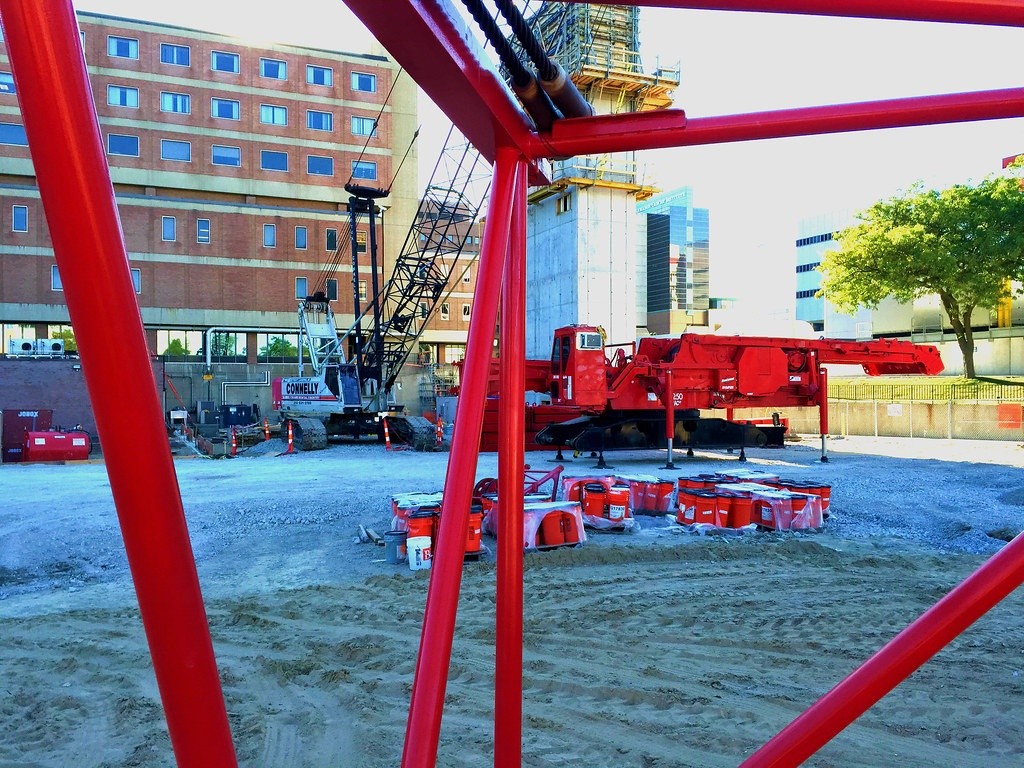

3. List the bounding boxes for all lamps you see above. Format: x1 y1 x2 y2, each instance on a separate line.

37 338 64 355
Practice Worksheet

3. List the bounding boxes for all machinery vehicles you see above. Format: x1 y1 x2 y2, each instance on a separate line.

454 321 945 471
217 404 266 445
272 0 607 452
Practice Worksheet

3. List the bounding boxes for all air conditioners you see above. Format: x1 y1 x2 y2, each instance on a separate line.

8 339 34 354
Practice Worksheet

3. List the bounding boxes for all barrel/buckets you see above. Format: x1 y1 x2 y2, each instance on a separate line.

384 531 407 563
404 536 432 570
390 468 832 553
